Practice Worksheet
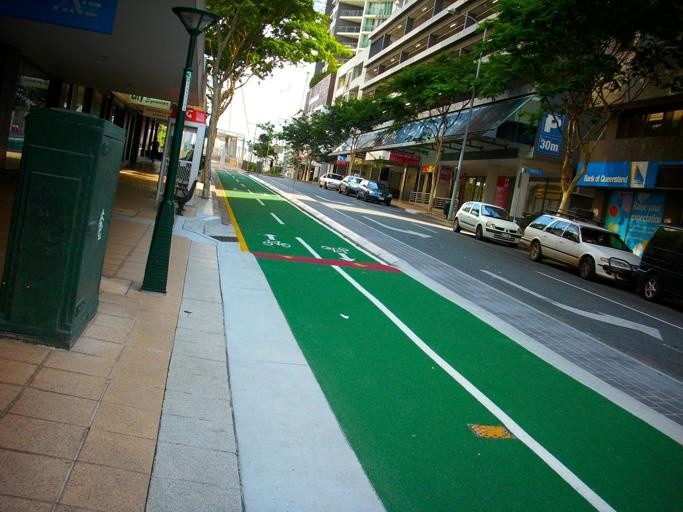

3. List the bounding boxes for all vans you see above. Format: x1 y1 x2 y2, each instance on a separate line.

636 223 683 310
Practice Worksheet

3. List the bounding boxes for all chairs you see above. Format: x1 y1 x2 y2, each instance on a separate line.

174 177 199 216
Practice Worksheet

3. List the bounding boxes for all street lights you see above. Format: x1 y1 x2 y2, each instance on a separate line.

443 7 488 220
137 4 226 296
245 122 258 171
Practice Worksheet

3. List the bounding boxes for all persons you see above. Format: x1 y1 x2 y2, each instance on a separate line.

150 137 159 163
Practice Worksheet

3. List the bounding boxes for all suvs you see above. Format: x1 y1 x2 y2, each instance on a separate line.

519 207 641 284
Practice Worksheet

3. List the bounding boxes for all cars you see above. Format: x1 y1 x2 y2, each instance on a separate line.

318 171 394 207
450 200 523 246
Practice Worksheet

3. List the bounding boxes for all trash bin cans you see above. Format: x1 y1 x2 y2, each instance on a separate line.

443 199 451 219
